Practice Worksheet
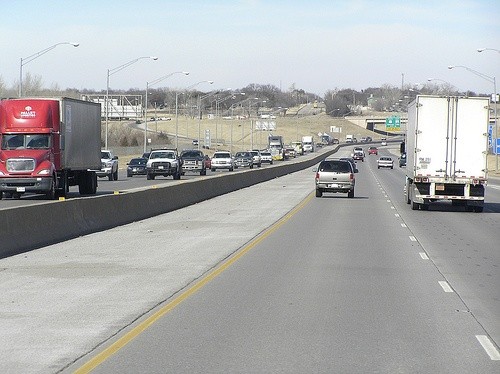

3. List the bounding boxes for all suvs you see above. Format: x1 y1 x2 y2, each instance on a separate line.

249 151 261 167
233 152 254 169
210 150 234 171
87 150 119 181
146 147 180 180
261 152 273 164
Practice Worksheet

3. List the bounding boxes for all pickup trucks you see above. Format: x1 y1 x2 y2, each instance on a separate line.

180 149 207 176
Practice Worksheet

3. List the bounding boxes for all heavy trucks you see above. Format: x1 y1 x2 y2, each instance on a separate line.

322 136 330 145
268 135 284 160
0 96 103 201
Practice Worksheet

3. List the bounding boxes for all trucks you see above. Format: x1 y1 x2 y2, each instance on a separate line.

302 136 314 152
404 94 491 213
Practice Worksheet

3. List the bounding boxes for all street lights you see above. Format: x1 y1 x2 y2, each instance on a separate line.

104 56 158 150
392 82 432 109
231 97 259 155
448 65 499 171
175 80 213 146
143 71 189 152
215 92 246 150
251 100 288 151
18 41 79 96
428 78 449 96
197 87 232 150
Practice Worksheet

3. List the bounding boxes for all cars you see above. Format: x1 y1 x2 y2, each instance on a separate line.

285 141 304 158
316 143 322 147
127 157 148 177
205 156 211 169
312 157 359 198
346 137 372 143
352 141 406 169
